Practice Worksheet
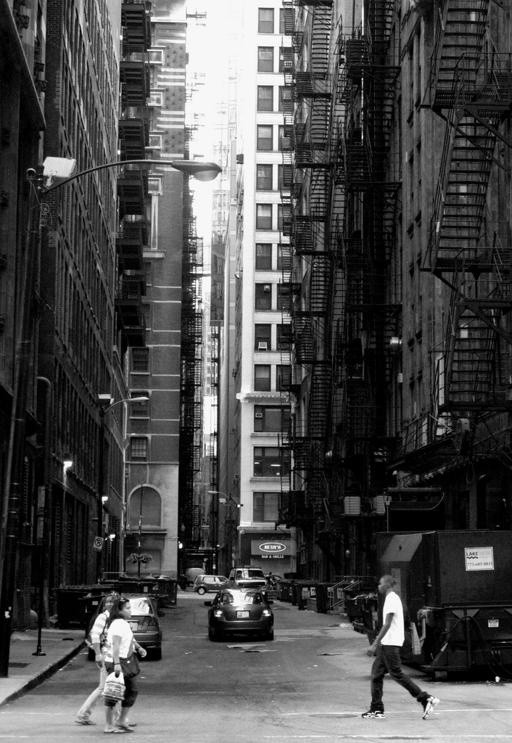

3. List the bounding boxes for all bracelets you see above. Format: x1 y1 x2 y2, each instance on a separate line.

136 646 142 651
114 663 120 665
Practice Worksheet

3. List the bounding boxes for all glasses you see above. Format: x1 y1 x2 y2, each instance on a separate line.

119 597 129 603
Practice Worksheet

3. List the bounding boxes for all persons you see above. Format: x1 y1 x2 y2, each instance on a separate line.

100 596 139 733
266 572 276 590
74 592 148 726
362 574 440 720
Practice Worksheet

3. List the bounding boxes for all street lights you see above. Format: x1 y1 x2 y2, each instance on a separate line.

96 396 150 584
208 490 230 518
0 160 221 674
269 463 323 497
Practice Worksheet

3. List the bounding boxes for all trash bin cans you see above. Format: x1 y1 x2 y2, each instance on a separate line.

315 583 328 612
56 586 118 630
181 576 187 589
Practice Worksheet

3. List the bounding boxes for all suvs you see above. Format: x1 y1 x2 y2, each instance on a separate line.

230 567 267 586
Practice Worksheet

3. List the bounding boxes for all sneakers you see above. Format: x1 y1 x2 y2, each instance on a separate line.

73 715 96 725
103 718 136 734
362 709 385 719
422 695 440 720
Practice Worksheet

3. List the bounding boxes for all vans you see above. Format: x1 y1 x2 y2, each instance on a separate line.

192 574 233 595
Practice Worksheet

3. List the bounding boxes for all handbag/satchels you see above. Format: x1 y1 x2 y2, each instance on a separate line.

119 653 140 679
86 632 106 662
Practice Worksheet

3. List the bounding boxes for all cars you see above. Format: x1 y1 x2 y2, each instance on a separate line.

208 587 274 643
85 592 163 662
